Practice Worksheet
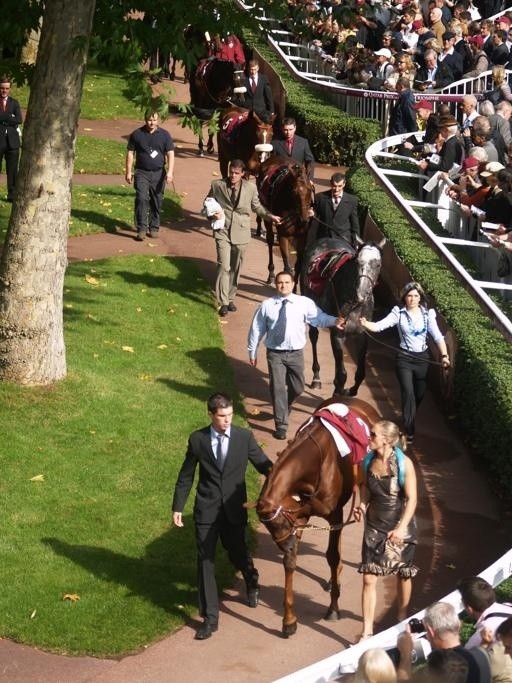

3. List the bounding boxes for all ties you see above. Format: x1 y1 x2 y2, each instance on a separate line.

230 188 237 206
2 98 6 112
216 435 225 470
335 196 339 203
250 77 257 93
288 141 291 155
270 299 287 345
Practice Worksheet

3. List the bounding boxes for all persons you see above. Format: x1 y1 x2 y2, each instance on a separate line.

349 421 419 650
172 395 276 639
232 53 274 124
127 109 175 241
335 576 512 680
208 32 246 70
202 159 282 317
302 173 359 252
1 78 23 202
270 119 315 209
247 271 347 440
253 0 512 124
359 281 452 445
388 78 512 306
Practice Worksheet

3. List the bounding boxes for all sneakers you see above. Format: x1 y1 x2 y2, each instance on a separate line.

407 434 414 444
274 428 287 440
151 231 158 238
137 231 146 241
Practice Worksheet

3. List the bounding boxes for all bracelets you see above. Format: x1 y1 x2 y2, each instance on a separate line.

441 353 449 359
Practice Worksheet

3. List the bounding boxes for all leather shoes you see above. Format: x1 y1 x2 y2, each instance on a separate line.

219 305 228 315
228 302 236 311
196 621 218 639
247 588 259 607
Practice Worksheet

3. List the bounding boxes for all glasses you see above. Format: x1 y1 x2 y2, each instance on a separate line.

370 431 377 438
382 37 391 41
398 61 406 64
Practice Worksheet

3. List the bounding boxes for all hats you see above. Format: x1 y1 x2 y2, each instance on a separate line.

495 16 511 25
409 20 424 33
410 100 505 178
442 31 456 40
373 48 391 59
468 35 484 47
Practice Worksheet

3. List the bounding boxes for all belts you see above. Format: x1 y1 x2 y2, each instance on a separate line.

267 348 299 353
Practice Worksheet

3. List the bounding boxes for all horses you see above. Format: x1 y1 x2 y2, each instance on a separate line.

243 396 382 637
189 57 246 157
254 153 314 284
217 106 277 181
300 231 388 397
